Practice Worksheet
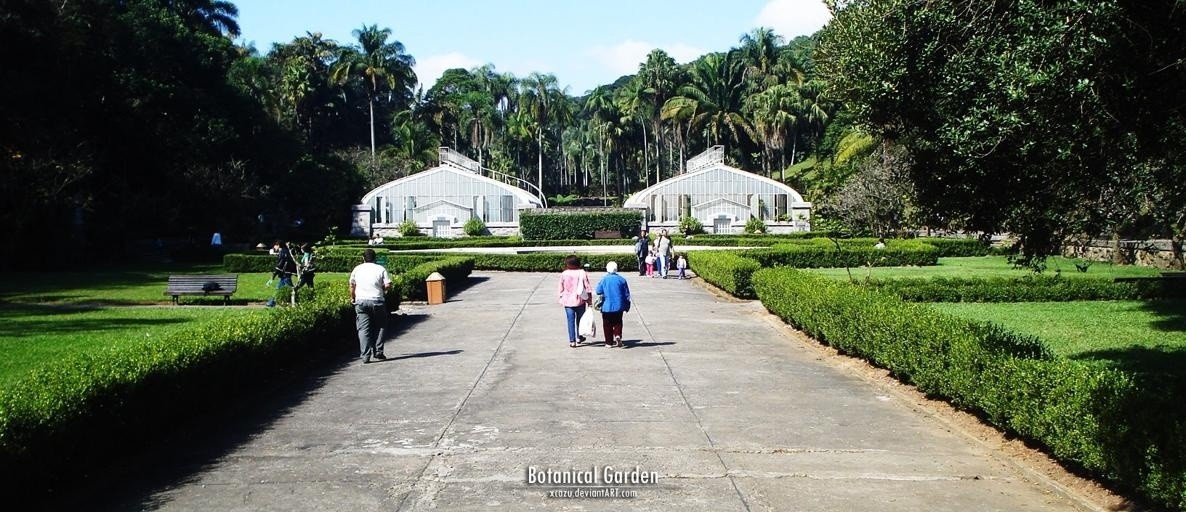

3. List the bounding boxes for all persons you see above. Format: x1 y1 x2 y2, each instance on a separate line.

595 260 631 349
558 254 592 348
873 238 886 247
366 233 384 246
263 239 302 307
292 243 316 301
675 252 686 280
682 225 695 239
347 249 393 365
631 229 675 279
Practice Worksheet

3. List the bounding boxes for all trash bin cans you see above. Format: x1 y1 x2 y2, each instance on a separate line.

425 272 446 305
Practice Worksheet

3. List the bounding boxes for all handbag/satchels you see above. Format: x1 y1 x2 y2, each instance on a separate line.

300 262 317 277
577 268 589 301
595 295 605 307
578 305 596 337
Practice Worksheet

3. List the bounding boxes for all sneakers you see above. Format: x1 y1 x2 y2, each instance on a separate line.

570 342 576 347
577 338 586 344
615 335 623 347
638 272 667 279
605 344 612 348
373 352 386 360
678 276 685 280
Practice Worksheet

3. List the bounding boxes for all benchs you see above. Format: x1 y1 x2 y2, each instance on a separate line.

164 271 238 305
